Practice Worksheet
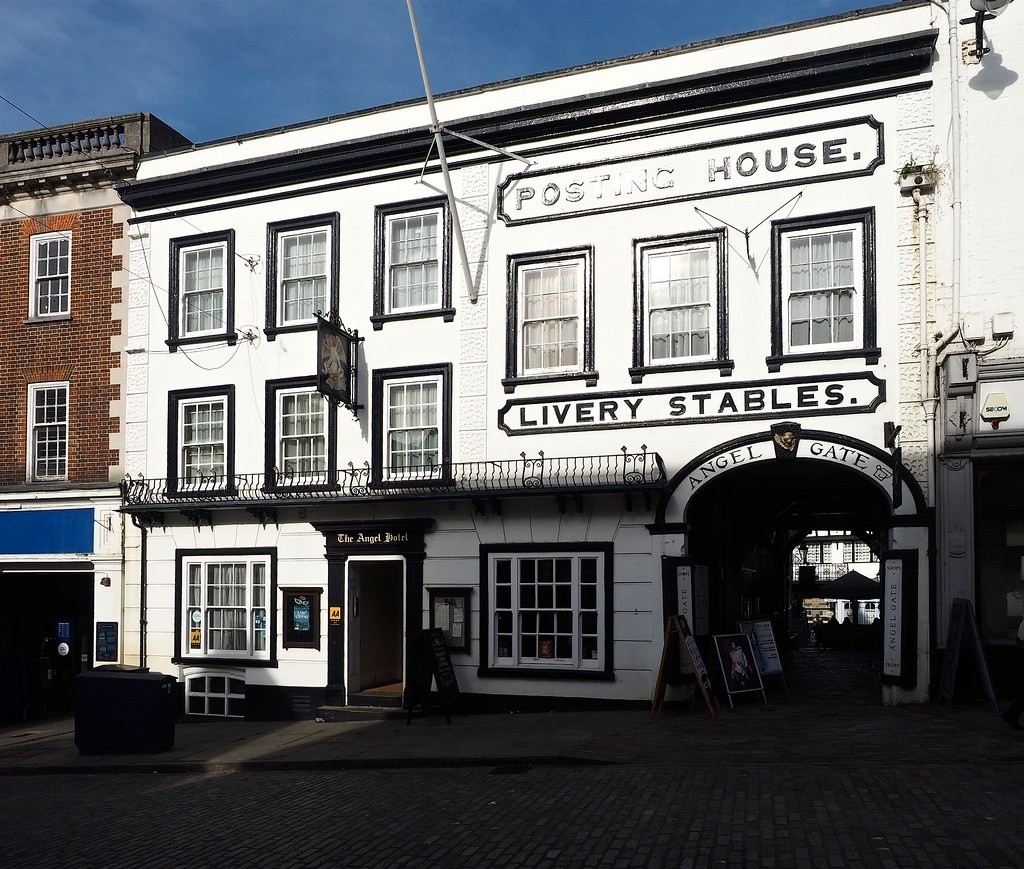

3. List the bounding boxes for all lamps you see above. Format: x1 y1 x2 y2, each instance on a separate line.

959 0 1015 66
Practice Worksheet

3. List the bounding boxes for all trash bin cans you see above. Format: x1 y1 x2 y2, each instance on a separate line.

75 670 177 755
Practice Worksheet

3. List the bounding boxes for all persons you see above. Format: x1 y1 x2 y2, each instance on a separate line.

813 614 822 623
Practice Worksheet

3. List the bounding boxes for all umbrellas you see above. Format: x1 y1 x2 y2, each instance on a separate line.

821 570 881 600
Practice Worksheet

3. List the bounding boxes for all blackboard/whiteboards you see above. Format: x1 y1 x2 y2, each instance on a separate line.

423 627 461 703
937 598 968 702
